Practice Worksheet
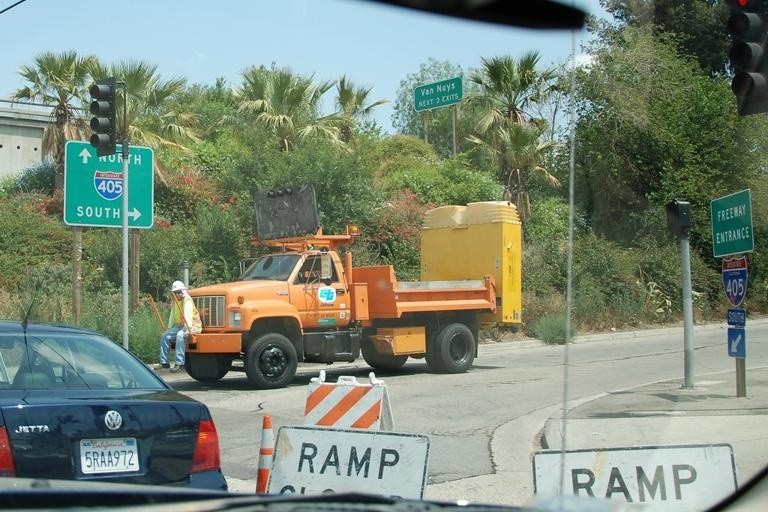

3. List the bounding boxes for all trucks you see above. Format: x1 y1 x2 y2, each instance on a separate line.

149 225 498 389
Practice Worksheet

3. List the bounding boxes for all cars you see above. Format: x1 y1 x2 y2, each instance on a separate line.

0 259 229 492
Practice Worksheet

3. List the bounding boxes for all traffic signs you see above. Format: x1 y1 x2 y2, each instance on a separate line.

722 254 747 307
712 189 754 257
63 141 154 231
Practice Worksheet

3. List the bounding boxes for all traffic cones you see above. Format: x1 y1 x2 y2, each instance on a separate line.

255 414 274 494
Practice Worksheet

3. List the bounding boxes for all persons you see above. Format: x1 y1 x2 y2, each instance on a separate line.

154 281 203 372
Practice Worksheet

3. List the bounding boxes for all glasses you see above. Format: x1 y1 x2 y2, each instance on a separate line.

174 291 181 295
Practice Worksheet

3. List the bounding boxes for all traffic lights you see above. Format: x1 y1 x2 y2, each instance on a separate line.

665 198 691 234
90 77 116 157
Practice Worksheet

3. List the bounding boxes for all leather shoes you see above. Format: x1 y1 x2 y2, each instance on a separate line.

170 364 183 373
154 363 170 370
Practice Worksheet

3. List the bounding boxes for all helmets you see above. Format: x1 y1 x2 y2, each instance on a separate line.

169 280 187 291
